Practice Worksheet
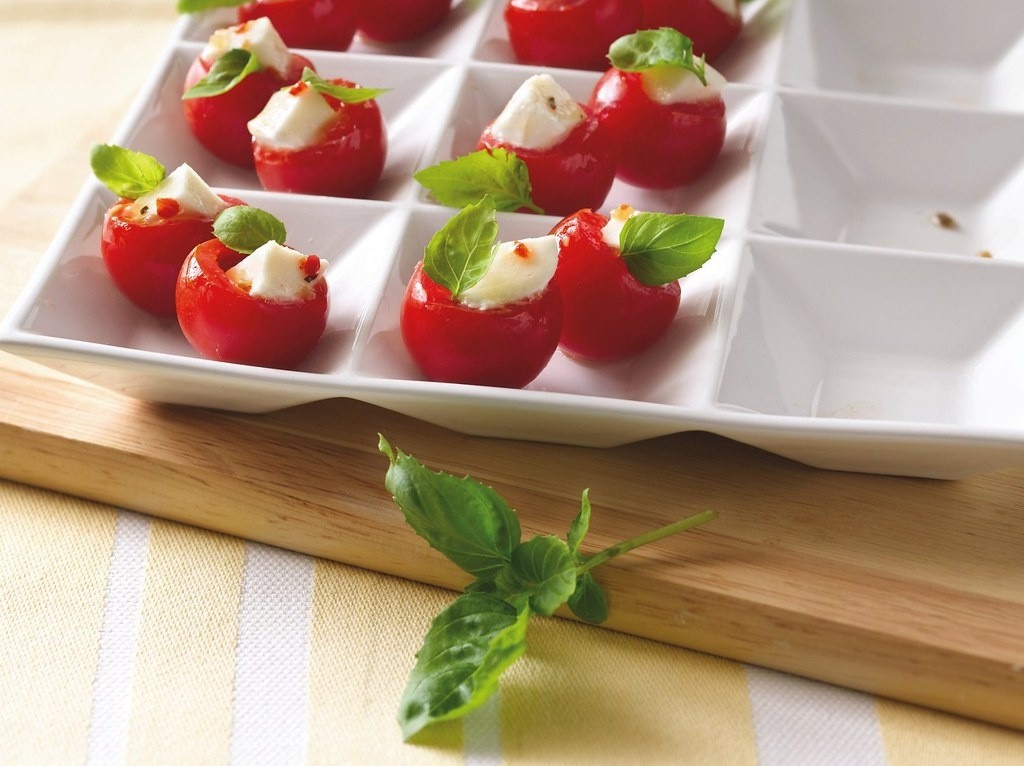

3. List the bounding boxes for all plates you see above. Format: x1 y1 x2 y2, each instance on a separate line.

0 0 1024 482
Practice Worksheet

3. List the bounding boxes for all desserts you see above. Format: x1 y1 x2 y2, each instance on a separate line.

88 0 746 391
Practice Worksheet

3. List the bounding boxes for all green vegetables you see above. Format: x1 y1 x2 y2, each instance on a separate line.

376 431 722 744
